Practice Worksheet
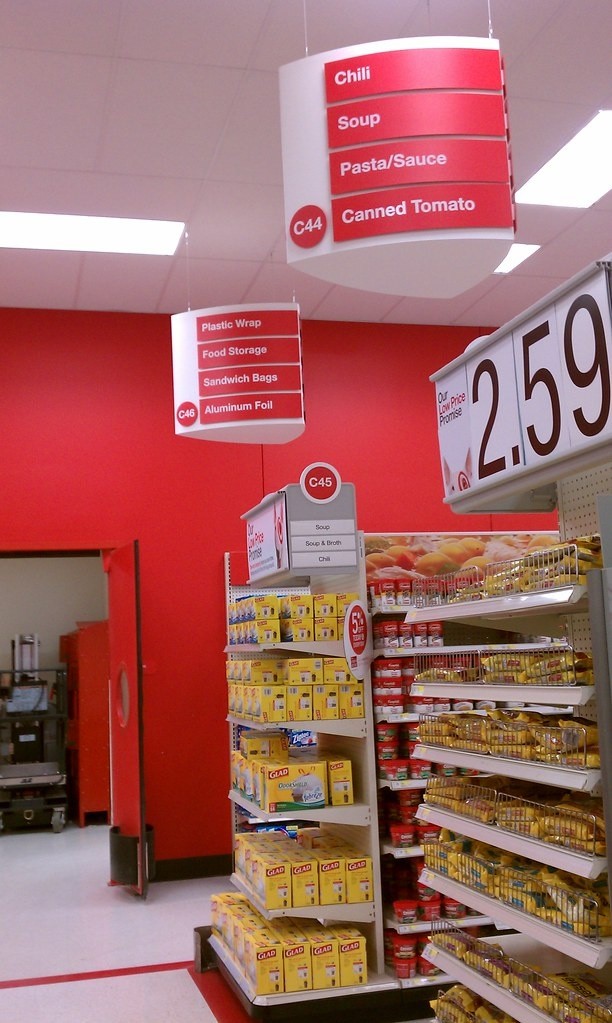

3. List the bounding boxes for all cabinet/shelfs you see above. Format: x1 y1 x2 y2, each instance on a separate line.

0 668 69 834
358 530 612 1023
59 619 111 829
206 549 402 1023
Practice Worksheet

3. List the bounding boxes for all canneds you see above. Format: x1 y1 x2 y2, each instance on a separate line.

372 659 490 981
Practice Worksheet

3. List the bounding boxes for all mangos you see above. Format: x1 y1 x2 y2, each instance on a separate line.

364 534 555 581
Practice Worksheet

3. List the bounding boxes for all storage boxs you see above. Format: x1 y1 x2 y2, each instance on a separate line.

235 828 375 910
228 592 361 644
224 656 365 724
230 731 353 814
6 678 48 713
211 893 368 996
